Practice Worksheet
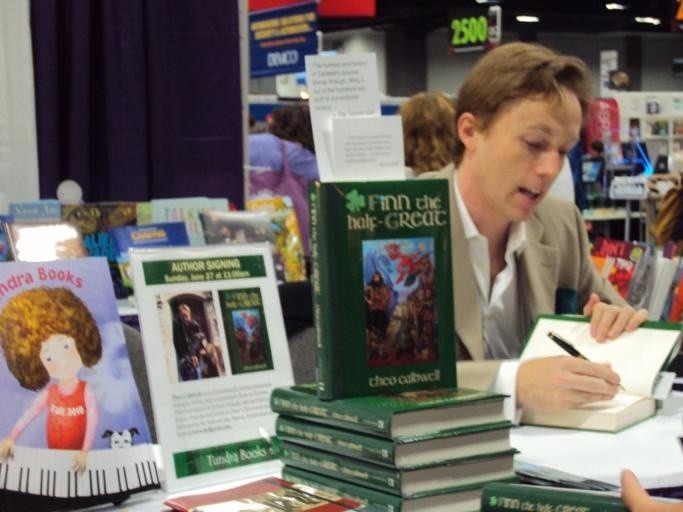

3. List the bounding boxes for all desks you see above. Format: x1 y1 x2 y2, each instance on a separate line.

74 489 173 512
582 209 646 242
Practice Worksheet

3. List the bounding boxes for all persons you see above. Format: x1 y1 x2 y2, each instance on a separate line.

394 90 465 182
269 104 315 156
415 41 650 409
565 96 589 232
581 140 603 160
247 115 320 339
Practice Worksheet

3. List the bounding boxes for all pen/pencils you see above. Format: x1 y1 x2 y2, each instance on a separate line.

547 332 626 392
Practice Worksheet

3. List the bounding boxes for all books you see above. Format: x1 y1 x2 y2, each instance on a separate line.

306 180 458 400
592 237 683 322
490 314 682 433
271 379 519 510
1 179 306 512
480 481 683 512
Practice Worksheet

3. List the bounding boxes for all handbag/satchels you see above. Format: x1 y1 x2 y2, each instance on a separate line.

252 167 311 255
